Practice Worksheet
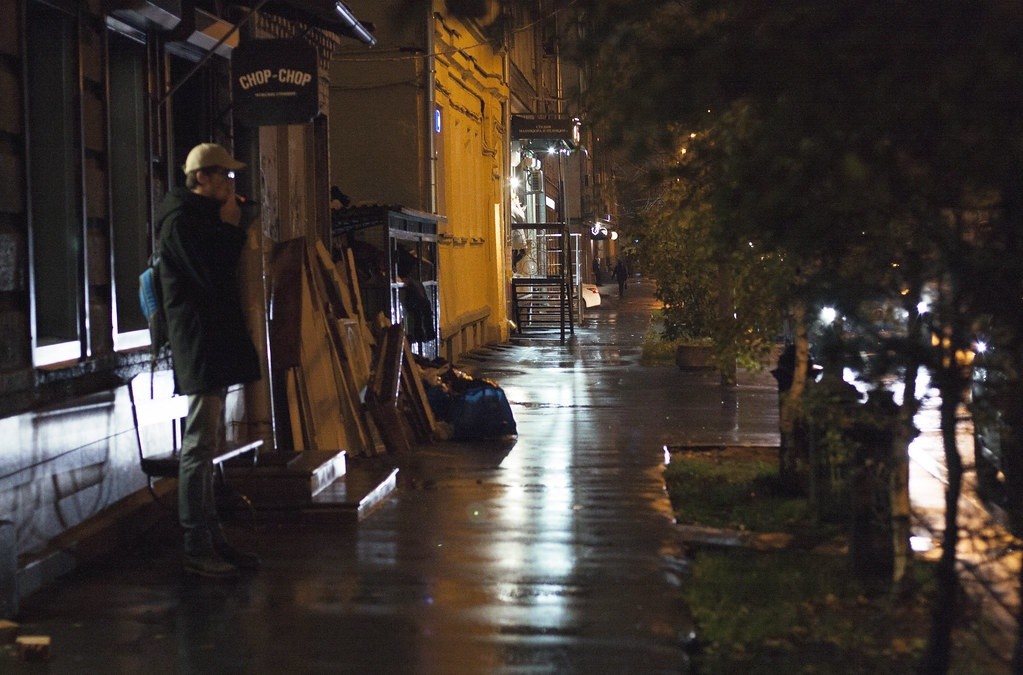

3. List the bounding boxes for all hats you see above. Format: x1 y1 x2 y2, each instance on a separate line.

185 143 247 171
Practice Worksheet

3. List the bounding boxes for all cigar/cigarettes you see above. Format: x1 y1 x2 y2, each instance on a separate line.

233 192 245 202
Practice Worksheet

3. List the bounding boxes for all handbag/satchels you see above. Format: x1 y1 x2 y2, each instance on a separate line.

139 268 170 357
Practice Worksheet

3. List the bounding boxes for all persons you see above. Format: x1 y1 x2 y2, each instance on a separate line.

593 256 602 286
511 193 527 278
612 260 627 296
154 143 262 580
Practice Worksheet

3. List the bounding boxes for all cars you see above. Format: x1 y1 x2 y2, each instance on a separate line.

545 283 601 313
804 282 924 362
973 317 1023 521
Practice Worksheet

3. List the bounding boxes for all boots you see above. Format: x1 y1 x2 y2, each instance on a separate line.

211 519 239 560
182 527 238 579
